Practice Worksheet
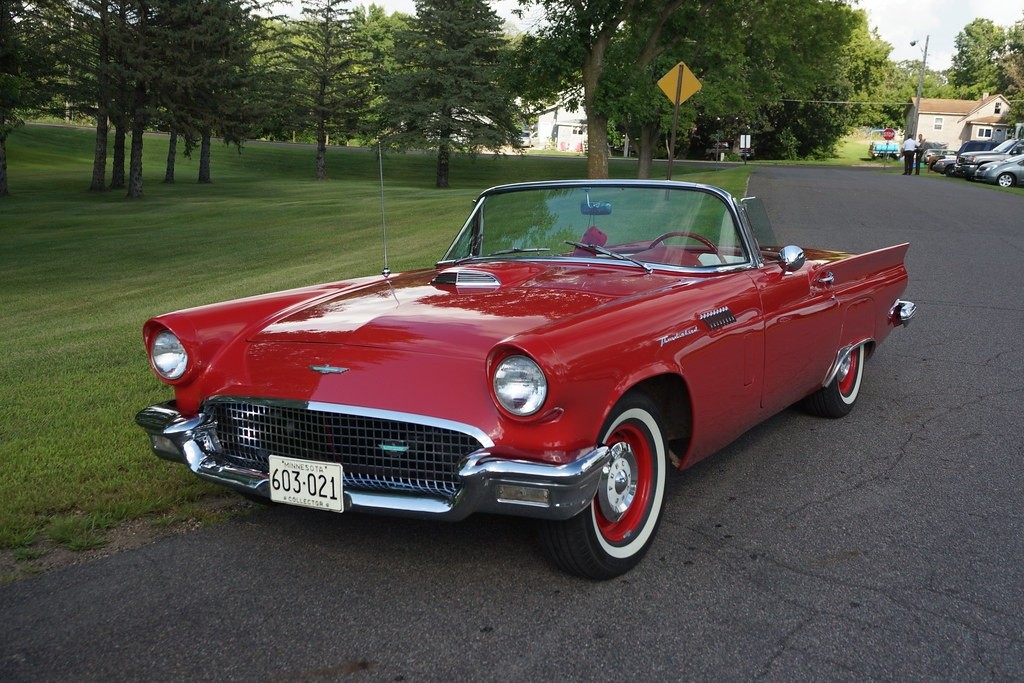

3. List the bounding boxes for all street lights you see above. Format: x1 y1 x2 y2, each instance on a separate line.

908 34 930 141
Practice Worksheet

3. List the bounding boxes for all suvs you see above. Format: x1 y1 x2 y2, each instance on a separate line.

953 137 1024 182
956 139 1001 160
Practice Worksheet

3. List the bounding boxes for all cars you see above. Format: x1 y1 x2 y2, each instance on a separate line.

974 153 1024 188
924 148 958 178
520 130 532 148
706 142 754 163
134 178 917 581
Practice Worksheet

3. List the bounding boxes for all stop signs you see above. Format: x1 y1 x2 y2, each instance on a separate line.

883 128 895 140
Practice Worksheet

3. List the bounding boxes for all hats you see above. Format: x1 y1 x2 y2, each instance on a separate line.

908 134 914 138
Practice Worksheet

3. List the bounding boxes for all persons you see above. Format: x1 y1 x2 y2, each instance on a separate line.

903 133 926 176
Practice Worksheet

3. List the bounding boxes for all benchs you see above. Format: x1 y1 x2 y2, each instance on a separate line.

597 248 746 266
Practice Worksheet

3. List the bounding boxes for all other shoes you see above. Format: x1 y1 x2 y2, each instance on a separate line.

909 173 912 175
902 173 907 175
